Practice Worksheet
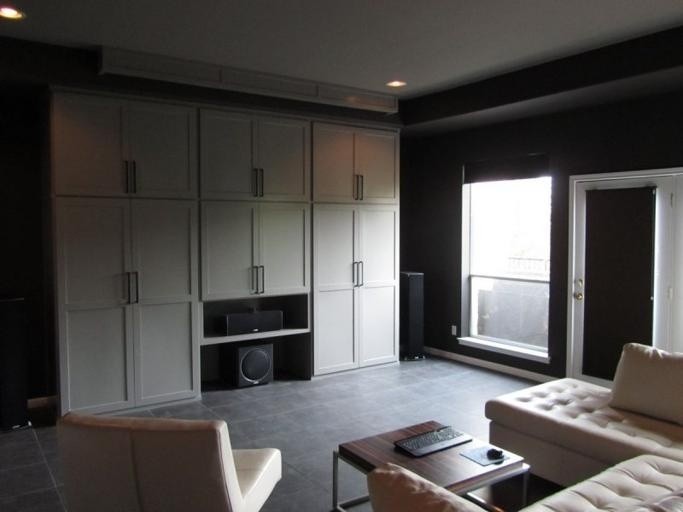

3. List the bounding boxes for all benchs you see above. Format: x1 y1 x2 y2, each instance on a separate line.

54 412 283 511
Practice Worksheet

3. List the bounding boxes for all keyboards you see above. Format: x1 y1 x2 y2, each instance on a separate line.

394 426 472 458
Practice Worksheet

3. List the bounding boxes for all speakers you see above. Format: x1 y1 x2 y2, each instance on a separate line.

401 270 426 360
214 309 283 336
0 303 32 430
219 342 273 388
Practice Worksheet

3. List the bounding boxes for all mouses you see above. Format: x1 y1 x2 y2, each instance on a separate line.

487 449 503 459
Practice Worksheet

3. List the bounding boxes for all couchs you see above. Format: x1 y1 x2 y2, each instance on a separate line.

366 376 683 511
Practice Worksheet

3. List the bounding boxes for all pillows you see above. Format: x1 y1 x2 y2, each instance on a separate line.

607 341 683 426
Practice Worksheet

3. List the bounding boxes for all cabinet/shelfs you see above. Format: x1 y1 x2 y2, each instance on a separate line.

48 83 406 418
